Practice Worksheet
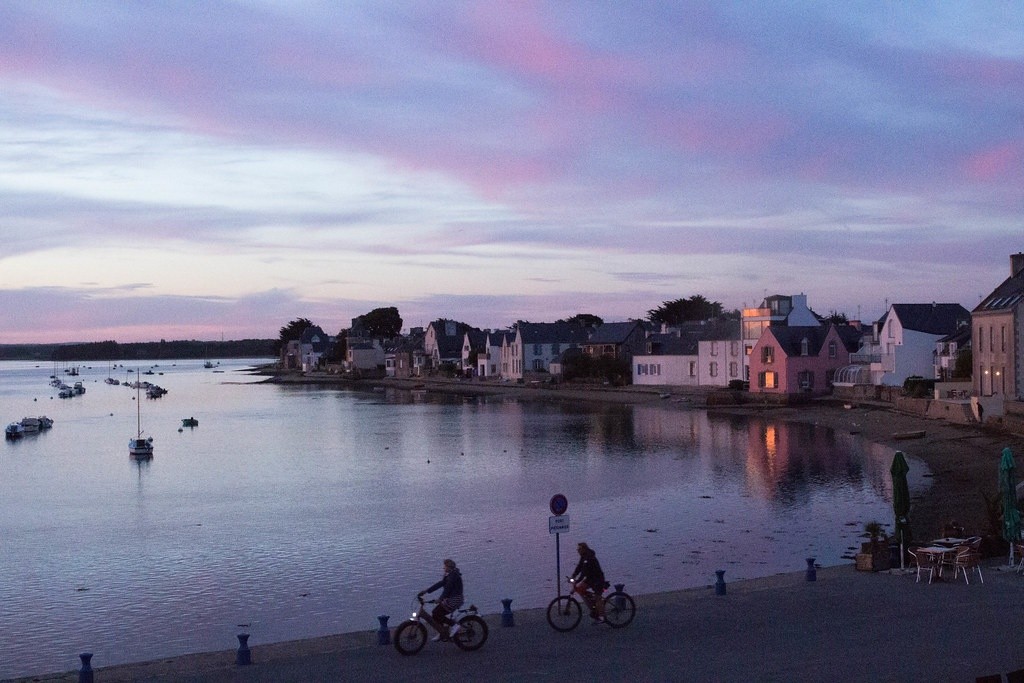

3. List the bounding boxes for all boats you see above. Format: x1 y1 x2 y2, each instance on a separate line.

142 369 154 374
181 416 199 428
4 415 54 439
204 347 218 368
113 380 168 399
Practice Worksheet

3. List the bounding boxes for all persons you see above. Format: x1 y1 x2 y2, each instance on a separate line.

567 542 605 623
418 559 462 642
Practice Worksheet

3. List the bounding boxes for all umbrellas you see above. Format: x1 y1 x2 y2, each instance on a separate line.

999 449 1021 566
890 452 912 570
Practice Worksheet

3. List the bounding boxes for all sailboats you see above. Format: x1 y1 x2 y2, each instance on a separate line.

50 353 86 399
128 366 154 454
104 360 114 383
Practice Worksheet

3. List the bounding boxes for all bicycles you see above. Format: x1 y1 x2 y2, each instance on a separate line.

546 576 636 632
394 596 488 656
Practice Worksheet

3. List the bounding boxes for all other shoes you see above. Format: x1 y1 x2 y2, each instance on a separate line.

449 624 460 637
591 617 606 625
432 633 441 641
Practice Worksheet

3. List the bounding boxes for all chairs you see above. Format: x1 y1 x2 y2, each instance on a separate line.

907 536 1024 587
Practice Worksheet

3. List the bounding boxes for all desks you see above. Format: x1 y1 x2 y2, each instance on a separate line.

917 547 955 575
